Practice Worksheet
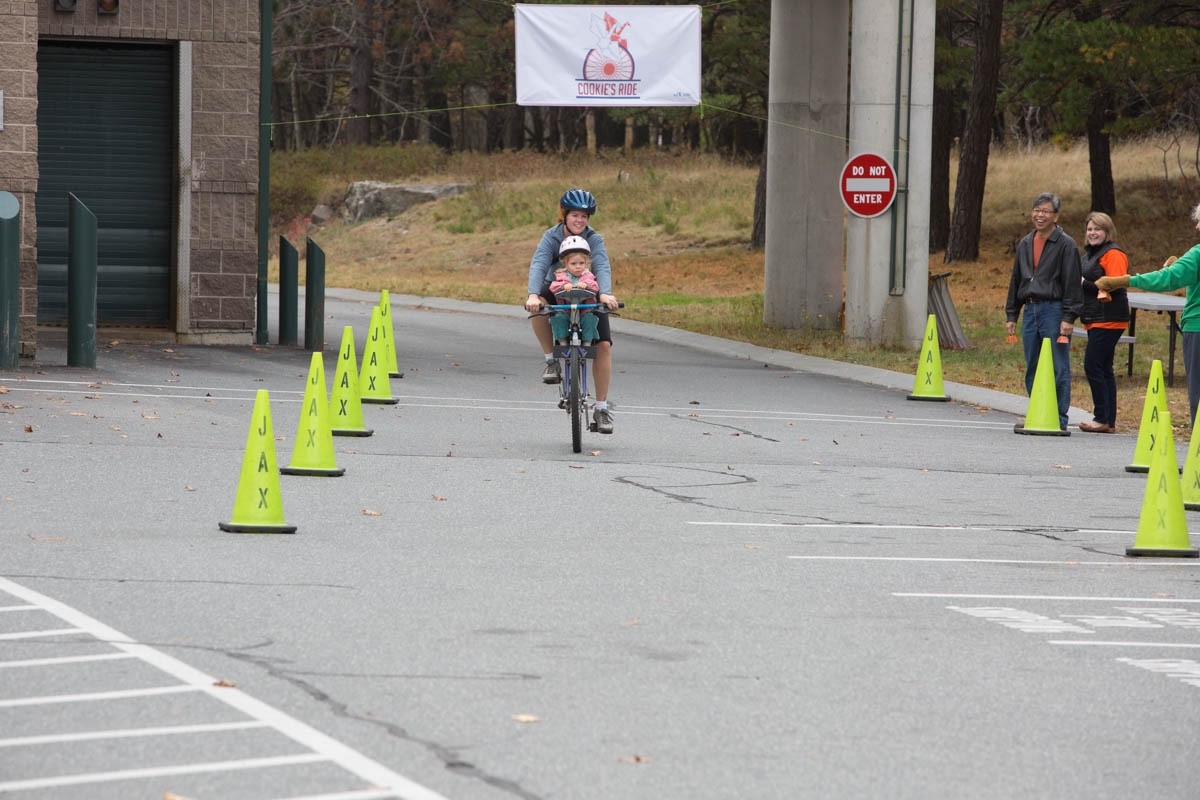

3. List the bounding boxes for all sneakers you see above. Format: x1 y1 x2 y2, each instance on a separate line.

593 408 614 433
541 359 561 383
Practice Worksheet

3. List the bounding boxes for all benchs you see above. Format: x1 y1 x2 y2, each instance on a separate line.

1072 327 1136 343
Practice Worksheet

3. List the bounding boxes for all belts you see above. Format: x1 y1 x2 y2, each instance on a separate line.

1025 296 1045 303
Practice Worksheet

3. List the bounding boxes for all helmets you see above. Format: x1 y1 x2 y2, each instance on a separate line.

558 235 591 258
559 189 596 210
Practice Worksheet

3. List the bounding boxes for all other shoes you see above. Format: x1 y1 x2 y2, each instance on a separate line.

1107 427 1116 433
1078 422 1109 432
1014 424 1025 434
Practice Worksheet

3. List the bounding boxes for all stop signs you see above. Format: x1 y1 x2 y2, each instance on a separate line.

839 153 899 218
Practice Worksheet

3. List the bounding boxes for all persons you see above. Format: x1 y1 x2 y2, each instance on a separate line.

549 234 598 362
1005 193 1081 431
1094 203 1200 434
526 189 620 435
1079 211 1129 434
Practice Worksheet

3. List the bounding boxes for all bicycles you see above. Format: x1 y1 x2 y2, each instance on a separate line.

518 296 627 452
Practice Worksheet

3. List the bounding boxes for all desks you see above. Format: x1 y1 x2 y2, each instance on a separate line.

1126 292 1186 387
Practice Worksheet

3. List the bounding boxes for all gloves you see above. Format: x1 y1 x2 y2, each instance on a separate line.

1094 275 1130 292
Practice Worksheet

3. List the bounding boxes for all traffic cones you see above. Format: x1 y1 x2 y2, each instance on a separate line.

324 322 374 436
1179 402 1200 511
1122 405 1200 557
1124 360 1187 476
371 287 406 378
1013 336 1074 437
356 305 400 403
907 311 952 403
217 387 302 536
276 351 348 477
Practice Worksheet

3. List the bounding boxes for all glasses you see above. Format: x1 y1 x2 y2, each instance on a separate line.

1032 208 1055 215
